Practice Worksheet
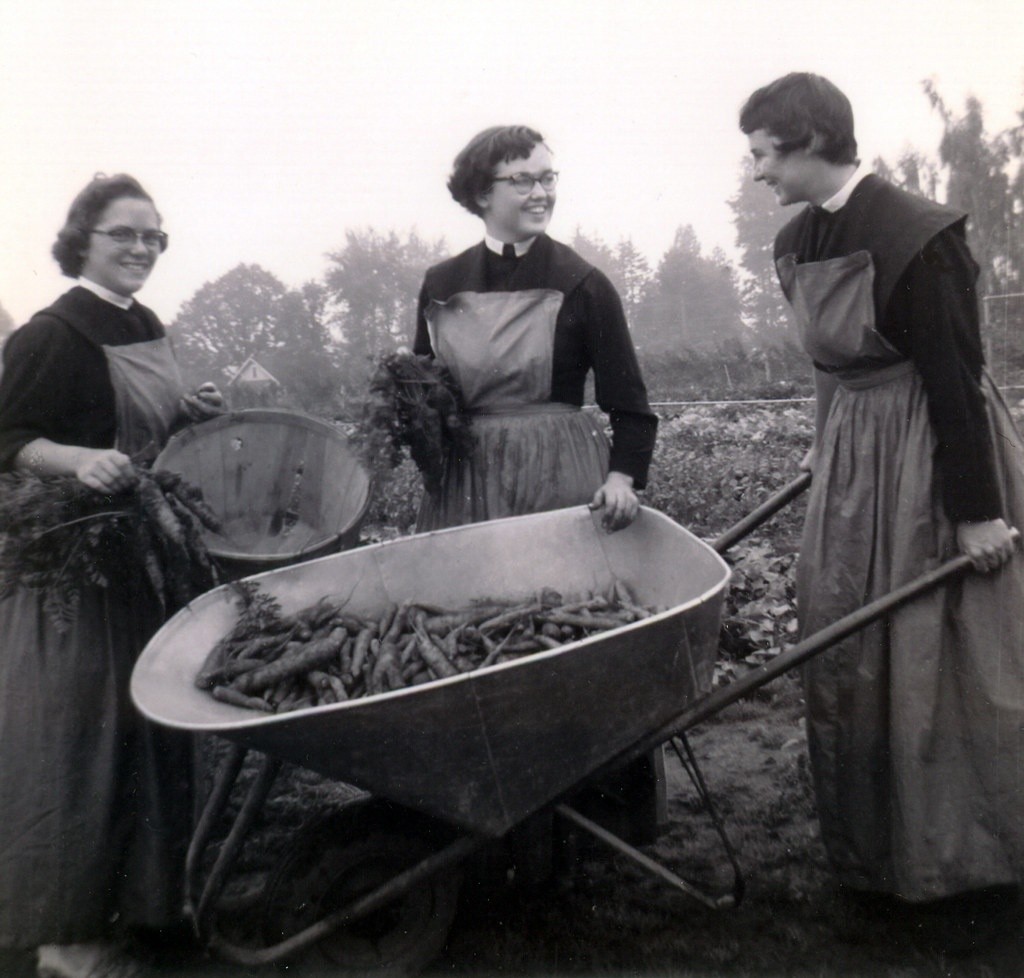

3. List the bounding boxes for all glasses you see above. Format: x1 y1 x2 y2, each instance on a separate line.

485 170 559 195
85 226 168 249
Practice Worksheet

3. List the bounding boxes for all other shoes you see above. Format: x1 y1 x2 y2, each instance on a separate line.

36 941 148 978
835 888 915 943
917 883 1023 955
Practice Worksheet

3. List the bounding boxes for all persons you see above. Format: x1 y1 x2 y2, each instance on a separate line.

377 124 661 924
739 74 1024 951
0 168 228 978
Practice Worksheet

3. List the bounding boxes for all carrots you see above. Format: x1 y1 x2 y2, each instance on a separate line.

194 578 659 713
0 467 221 614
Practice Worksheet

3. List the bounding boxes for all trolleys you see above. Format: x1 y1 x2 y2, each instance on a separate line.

122 469 1022 967
